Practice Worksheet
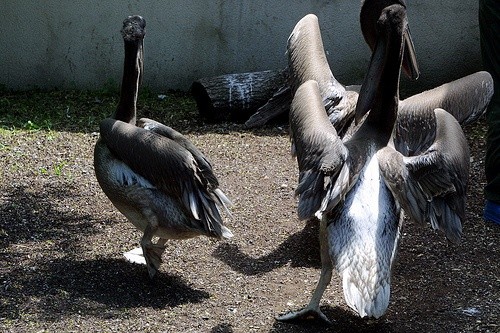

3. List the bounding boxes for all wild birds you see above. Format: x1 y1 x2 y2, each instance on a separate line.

273 4 474 324
286 0 493 157
93 15 238 281
